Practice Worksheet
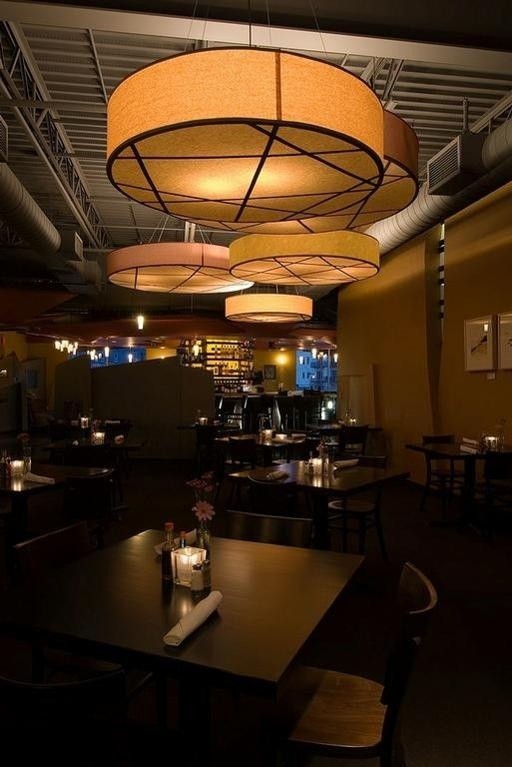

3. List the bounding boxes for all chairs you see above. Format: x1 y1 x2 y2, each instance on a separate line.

193 394 512 561
0 668 128 767
12 521 167 742
277 563 438 767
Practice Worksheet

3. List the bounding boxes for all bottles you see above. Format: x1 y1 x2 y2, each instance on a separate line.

304 462 314 475
259 430 266 444
0 456 13 480
161 522 187 584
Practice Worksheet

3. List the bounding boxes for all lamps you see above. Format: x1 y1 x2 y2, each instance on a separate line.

229 230 381 286
170 109 420 235
105 0 383 222
225 285 313 323
105 213 254 294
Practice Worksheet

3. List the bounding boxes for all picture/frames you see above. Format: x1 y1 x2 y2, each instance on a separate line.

497 311 512 370
463 313 497 371
264 364 276 380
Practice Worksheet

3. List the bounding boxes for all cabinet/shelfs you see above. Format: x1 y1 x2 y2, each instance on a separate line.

179 336 251 380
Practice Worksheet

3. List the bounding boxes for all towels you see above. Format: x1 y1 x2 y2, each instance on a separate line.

26 472 55 484
163 591 221 646
